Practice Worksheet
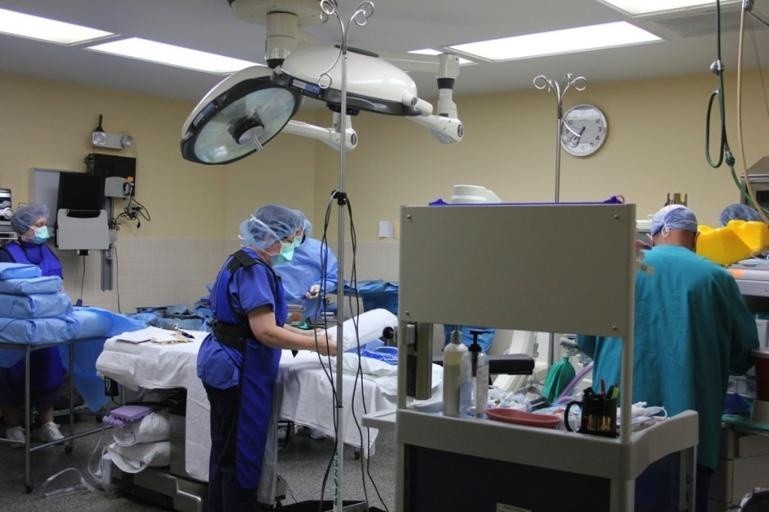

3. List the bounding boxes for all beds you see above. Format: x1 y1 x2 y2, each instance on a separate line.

97 297 493 510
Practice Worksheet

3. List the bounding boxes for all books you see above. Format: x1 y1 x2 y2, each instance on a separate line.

315 305 397 355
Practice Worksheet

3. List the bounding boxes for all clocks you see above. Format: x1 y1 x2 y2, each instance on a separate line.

557 102 609 158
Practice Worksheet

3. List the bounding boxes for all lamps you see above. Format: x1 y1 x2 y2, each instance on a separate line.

89 128 134 149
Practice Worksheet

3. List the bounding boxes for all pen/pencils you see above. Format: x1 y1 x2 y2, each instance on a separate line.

600 378 619 400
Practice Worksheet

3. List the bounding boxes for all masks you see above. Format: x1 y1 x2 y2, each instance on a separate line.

269 232 295 261
294 234 303 248
28 226 50 245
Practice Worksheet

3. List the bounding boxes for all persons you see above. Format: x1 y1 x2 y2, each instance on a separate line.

579 203 758 509
4 199 68 447
198 206 339 511
270 219 339 325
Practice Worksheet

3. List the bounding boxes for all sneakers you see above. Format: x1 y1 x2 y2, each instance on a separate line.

35 421 65 445
4 425 26 448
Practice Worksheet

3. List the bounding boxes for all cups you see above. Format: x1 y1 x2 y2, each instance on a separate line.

564 392 617 437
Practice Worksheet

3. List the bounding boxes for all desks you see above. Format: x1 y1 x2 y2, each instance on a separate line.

0 310 123 490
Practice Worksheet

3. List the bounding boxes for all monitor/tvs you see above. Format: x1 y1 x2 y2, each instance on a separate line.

745 183 769 215
0 188 12 227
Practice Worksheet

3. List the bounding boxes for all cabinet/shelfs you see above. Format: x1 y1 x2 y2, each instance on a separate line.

359 204 701 511
698 160 769 453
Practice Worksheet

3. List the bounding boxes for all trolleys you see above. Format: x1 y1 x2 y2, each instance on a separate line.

1 333 128 496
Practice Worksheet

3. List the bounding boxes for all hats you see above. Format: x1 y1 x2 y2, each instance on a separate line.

650 204 698 237
719 203 764 227
289 208 312 235
10 201 50 235
239 203 305 250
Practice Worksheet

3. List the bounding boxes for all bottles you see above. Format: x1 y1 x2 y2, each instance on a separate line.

458 331 490 417
442 326 471 417
